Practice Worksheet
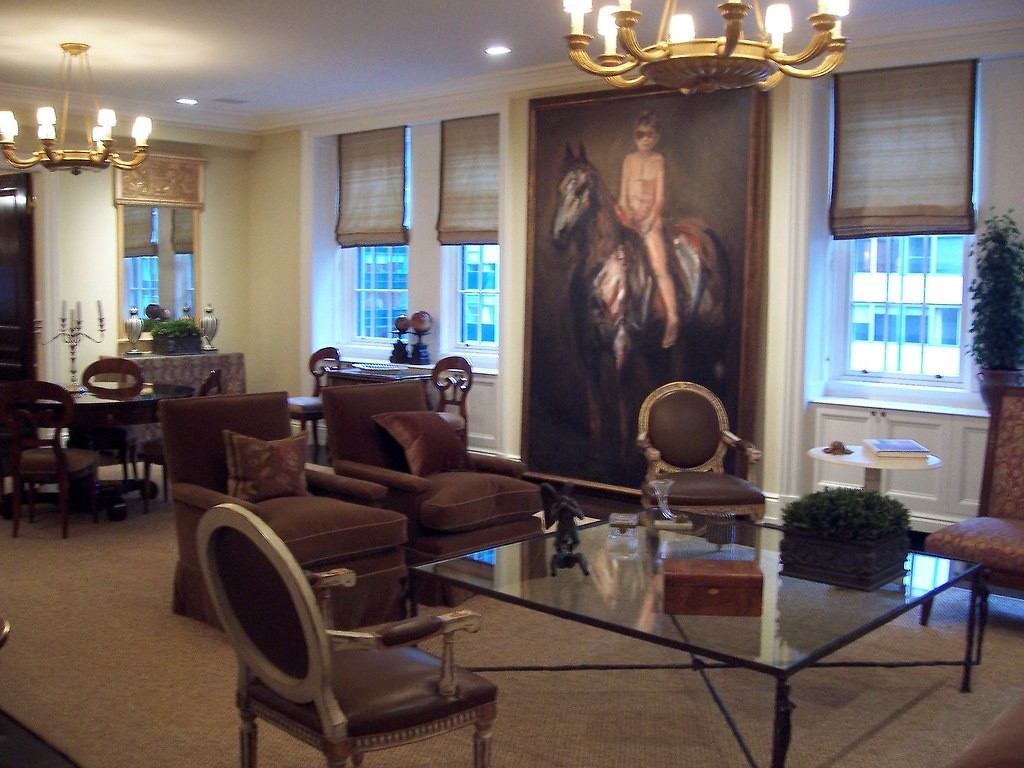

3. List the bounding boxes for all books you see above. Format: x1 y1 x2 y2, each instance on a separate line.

866 440 930 458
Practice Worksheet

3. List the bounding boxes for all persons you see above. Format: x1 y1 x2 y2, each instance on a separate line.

550 495 590 577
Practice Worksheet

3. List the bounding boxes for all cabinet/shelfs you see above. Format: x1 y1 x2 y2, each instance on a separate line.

810 403 954 533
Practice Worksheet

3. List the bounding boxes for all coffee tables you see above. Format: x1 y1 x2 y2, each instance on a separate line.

406 507 988 768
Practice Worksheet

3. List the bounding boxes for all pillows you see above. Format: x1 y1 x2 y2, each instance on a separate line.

223 429 311 502
371 411 476 478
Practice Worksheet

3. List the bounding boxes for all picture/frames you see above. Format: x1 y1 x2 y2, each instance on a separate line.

520 80 772 520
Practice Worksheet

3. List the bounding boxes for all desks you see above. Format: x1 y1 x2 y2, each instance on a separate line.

8 381 196 526
326 367 434 387
807 444 945 498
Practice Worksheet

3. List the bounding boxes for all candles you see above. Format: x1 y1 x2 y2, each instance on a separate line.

34 299 105 328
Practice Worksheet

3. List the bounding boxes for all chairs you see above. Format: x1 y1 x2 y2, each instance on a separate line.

194 503 503 768
634 380 767 573
919 387 1024 629
286 347 473 466
0 357 225 540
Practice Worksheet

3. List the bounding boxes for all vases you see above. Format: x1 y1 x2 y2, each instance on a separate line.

200 304 218 351
125 307 143 355
179 306 193 320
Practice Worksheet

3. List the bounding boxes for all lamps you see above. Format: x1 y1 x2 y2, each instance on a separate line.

562 0 853 97
0 41 153 177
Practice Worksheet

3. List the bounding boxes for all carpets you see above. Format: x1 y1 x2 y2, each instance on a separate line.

0 460 1024 768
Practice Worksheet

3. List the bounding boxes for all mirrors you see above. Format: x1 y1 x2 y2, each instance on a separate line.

117 203 201 342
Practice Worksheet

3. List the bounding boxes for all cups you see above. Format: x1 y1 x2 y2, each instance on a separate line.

604 512 641 560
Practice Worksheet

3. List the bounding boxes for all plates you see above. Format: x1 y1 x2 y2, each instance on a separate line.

351 363 410 374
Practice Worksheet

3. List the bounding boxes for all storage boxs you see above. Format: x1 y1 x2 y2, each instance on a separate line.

658 555 766 618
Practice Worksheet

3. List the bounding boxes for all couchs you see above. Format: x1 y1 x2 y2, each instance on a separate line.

159 391 406 637
319 376 549 611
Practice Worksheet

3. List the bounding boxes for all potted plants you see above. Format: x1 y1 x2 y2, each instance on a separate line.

778 484 913 575
970 203 1024 413
152 317 202 355
